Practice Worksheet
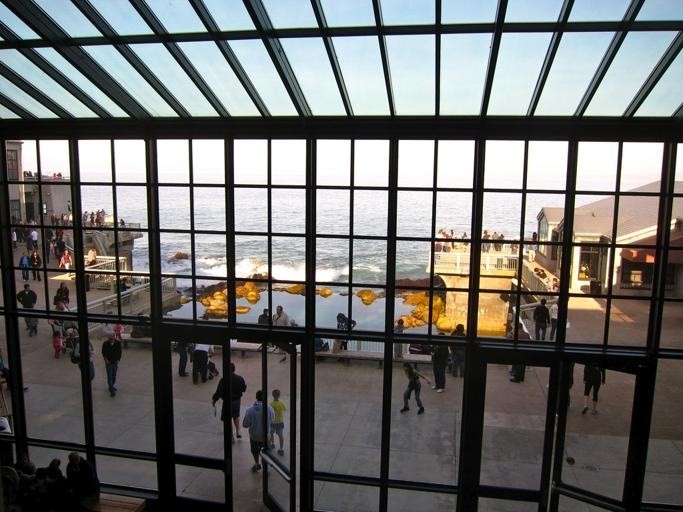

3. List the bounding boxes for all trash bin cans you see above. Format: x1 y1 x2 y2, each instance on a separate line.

86 275 90 291
590 280 601 294
0 415 13 464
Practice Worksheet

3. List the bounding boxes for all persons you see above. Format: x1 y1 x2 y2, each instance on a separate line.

176 298 289 475
395 319 466 414
0 451 100 512
0 170 125 398
582 365 605 415
556 362 576 419
532 268 562 341
438 229 538 262
510 324 531 384
340 317 356 350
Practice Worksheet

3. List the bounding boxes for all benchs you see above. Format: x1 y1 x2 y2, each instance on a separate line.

91 493 147 512
315 349 433 370
117 333 262 357
